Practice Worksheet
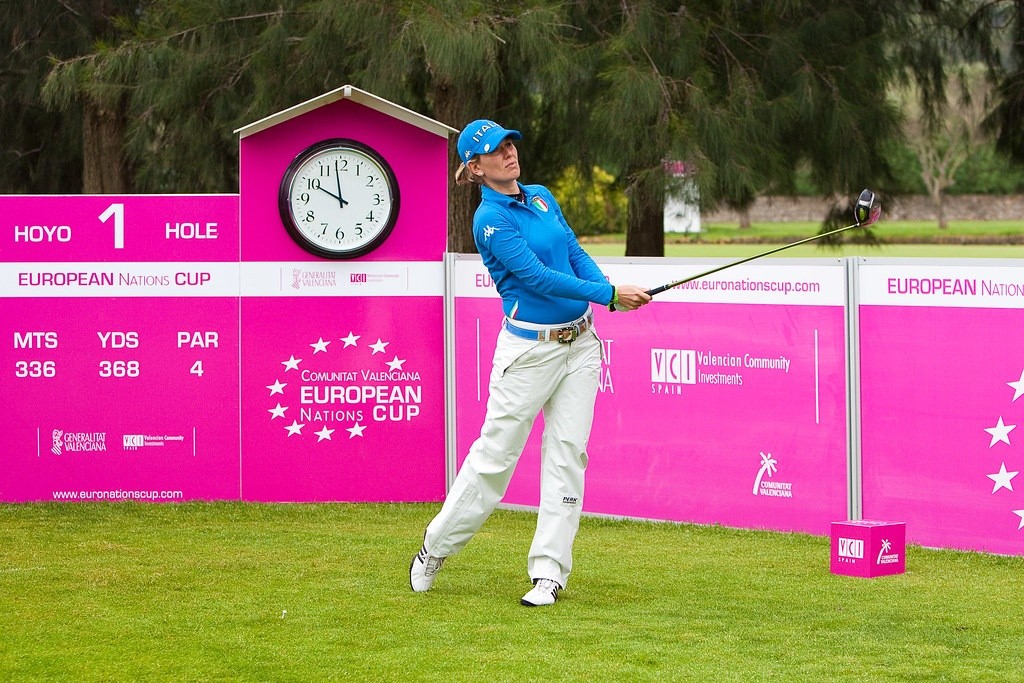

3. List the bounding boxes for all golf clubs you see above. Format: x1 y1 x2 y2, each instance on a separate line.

607 187 883 313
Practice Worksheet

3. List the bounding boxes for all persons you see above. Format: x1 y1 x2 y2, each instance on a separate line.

410 118 652 606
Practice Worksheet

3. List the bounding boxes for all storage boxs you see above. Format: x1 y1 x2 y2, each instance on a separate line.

831 519 906 578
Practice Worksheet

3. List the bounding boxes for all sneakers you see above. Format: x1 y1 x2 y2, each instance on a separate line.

521 579 559 606
410 526 446 591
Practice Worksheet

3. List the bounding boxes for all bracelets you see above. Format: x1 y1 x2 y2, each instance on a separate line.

609 286 619 303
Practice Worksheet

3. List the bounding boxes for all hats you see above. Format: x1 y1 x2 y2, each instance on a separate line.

457 118 522 166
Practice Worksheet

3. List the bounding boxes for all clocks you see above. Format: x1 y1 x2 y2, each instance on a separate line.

279 138 401 260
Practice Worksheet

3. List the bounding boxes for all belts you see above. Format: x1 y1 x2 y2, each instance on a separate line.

506 309 594 344
